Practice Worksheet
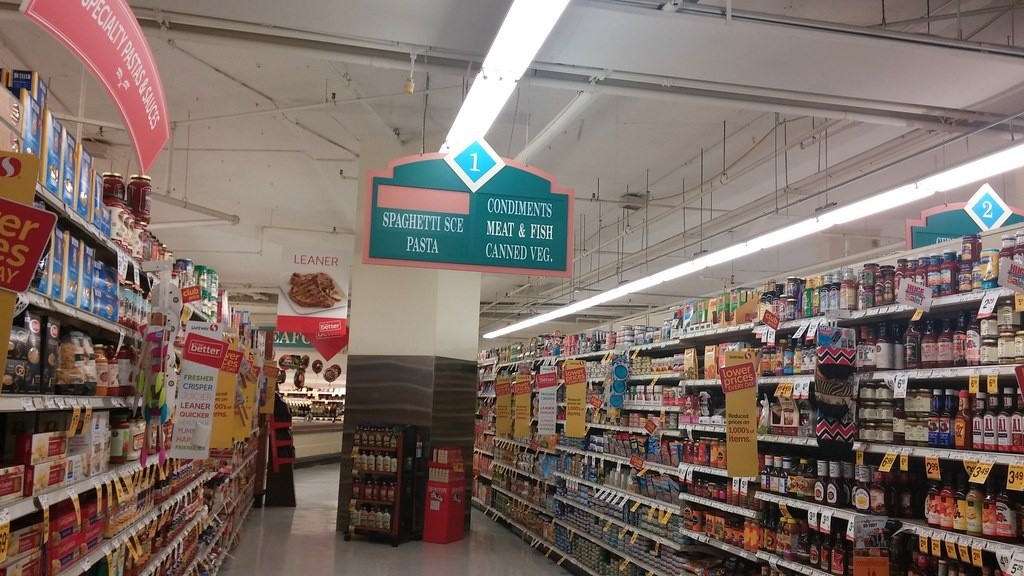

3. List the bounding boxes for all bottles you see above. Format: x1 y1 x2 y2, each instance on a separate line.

416 434 422 458
760 454 1024 544
745 334 817 376
117 343 133 395
490 489 555 544
858 381 1024 454
681 504 1004 576
855 298 1024 373
127 416 141 462
118 279 150 331
562 332 590 357
406 457 413 472
286 401 337 417
108 459 203 576
352 425 399 530
687 478 727 503
102 172 175 280
110 413 130 464
93 344 109 396
683 437 727 469
104 345 120 396
137 415 146 448
757 230 1024 325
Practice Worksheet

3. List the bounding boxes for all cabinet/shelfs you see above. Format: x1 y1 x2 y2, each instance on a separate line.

344 422 430 549
473 219 1024 576
278 387 346 422
1 65 299 576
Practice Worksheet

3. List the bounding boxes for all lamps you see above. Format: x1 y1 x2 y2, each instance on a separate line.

482 106 1024 340
